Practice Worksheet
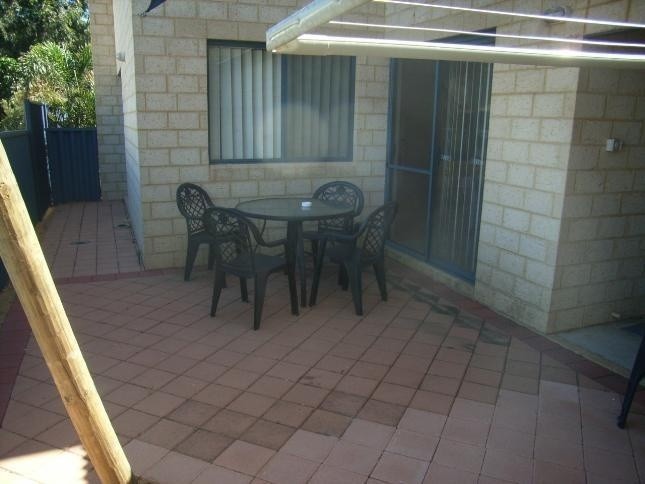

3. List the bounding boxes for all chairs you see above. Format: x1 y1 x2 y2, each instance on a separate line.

176 181 399 330
